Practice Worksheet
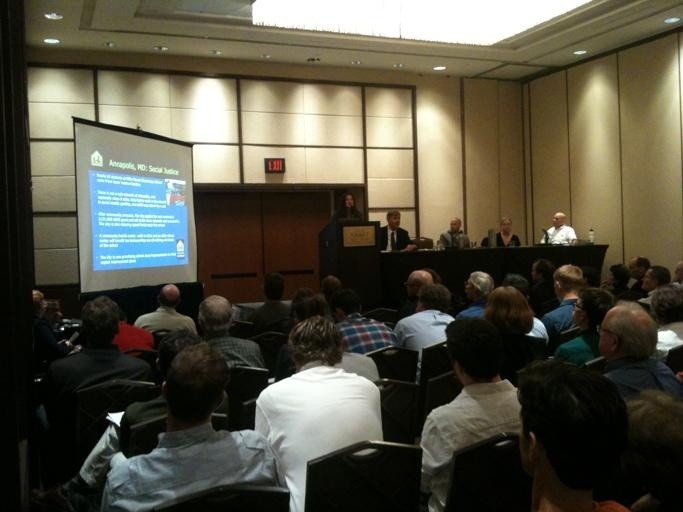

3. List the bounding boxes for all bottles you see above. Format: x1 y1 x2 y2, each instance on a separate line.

588 227 595 244
456 230 464 249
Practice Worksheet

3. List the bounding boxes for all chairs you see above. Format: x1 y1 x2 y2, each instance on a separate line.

411 237 433 249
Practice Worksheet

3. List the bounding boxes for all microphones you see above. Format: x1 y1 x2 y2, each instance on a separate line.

542 228 549 235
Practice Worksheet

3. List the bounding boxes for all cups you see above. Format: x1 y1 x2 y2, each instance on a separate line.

436 240 442 250
470 240 476 248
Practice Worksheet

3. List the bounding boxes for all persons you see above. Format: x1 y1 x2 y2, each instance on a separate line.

394 256 683 512
27 271 402 511
439 217 470 251
381 209 416 251
330 192 361 219
480 214 522 248
537 210 578 245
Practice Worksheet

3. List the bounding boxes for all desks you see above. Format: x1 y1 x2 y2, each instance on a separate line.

378 243 609 289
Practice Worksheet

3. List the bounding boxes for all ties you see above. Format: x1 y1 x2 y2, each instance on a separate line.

391 232 397 251
